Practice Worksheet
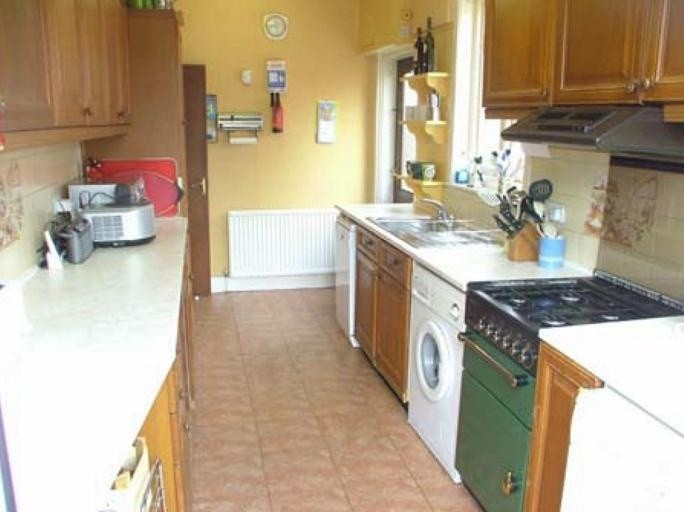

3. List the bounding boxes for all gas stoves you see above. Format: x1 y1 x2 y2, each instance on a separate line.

480 275 666 329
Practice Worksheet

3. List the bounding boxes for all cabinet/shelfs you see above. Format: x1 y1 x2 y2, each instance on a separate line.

141 231 196 512
354 227 412 412
522 340 603 512
0 0 131 150
397 71 450 213
480 0 684 130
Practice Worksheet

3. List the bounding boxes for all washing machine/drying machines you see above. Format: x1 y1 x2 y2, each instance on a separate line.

409 265 466 486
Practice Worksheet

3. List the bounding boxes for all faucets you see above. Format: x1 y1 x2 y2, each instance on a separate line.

420 197 448 220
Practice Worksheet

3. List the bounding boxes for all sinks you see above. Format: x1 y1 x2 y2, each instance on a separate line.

369 216 453 248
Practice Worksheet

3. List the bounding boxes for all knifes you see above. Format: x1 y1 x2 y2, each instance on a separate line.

492 209 521 236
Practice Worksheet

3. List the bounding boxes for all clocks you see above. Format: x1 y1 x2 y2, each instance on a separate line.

263 13 288 41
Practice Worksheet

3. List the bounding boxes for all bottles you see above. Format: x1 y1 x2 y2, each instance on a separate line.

413 15 436 75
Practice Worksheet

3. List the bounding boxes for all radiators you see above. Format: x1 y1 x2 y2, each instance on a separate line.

227 208 338 278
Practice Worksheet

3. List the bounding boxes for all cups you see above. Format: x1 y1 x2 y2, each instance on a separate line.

405 105 430 122
406 160 437 183
536 236 567 270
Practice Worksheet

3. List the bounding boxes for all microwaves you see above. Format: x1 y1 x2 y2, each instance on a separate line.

67 172 145 204
